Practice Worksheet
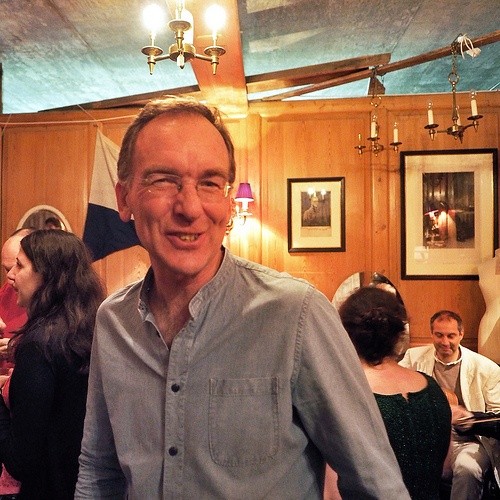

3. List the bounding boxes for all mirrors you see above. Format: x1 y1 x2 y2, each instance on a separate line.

15 205 73 233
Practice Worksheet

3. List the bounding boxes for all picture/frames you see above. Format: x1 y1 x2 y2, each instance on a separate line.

400 148 499 280
287 177 345 253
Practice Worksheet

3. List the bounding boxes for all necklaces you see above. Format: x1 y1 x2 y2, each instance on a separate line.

434 356 462 366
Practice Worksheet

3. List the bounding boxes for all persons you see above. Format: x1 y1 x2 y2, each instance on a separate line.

398 310 500 500
0 226 107 500
46 217 61 230
323 287 452 500
73 98 412 500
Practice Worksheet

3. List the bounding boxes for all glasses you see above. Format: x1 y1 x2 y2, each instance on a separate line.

126 168 234 203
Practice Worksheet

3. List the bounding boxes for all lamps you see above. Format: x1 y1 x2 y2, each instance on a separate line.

233 182 254 223
424 34 483 144
355 65 402 155
141 0 226 75
